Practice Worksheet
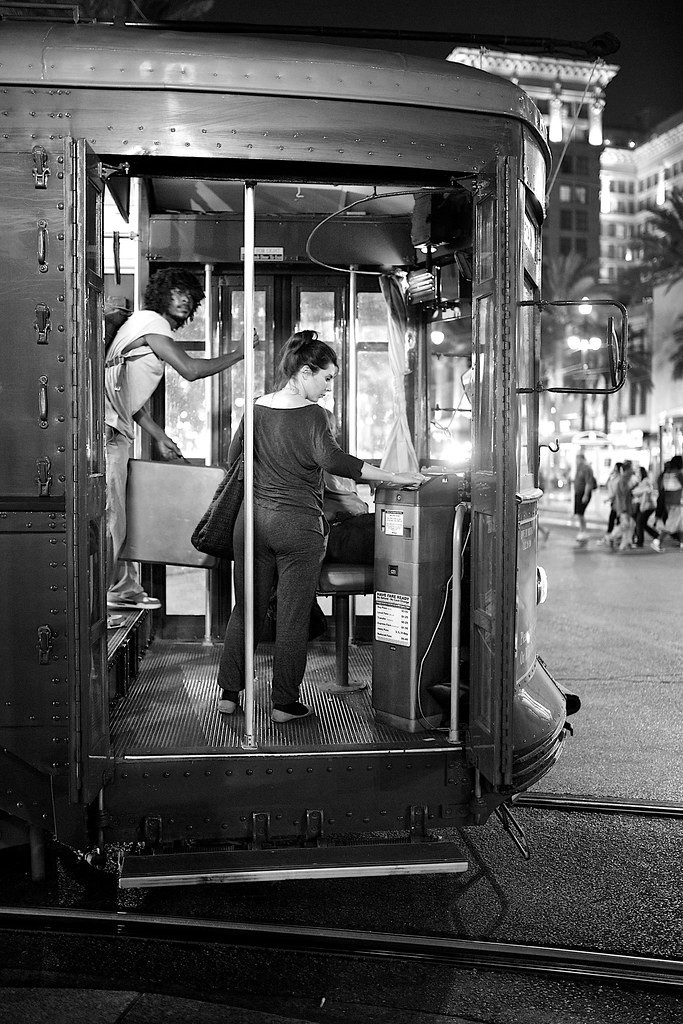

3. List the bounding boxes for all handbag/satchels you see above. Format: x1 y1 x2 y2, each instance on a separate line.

258 562 330 643
190 416 243 561
640 490 658 513
592 476 601 490
323 510 376 566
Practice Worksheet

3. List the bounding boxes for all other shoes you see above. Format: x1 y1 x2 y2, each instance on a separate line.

604 535 615 552
620 544 631 551
576 531 590 541
542 528 549 543
680 543 683 547
270 703 314 722
218 692 240 713
631 543 643 549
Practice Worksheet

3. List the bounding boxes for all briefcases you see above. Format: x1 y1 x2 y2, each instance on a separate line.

119 452 229 568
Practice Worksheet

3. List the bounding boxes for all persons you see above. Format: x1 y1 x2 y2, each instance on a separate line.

104 267 260 629
597 455 683 554
572 454 593 543
217 331 421 723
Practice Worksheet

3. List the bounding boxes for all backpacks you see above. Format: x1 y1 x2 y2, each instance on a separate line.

662 472 683 492
106 305 132 370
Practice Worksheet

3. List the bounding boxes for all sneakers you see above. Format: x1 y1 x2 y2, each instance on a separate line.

106 590 162 608
650 540 666 553
107 613 128 629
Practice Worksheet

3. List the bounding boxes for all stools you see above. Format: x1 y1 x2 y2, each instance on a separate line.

318 564 376 693
